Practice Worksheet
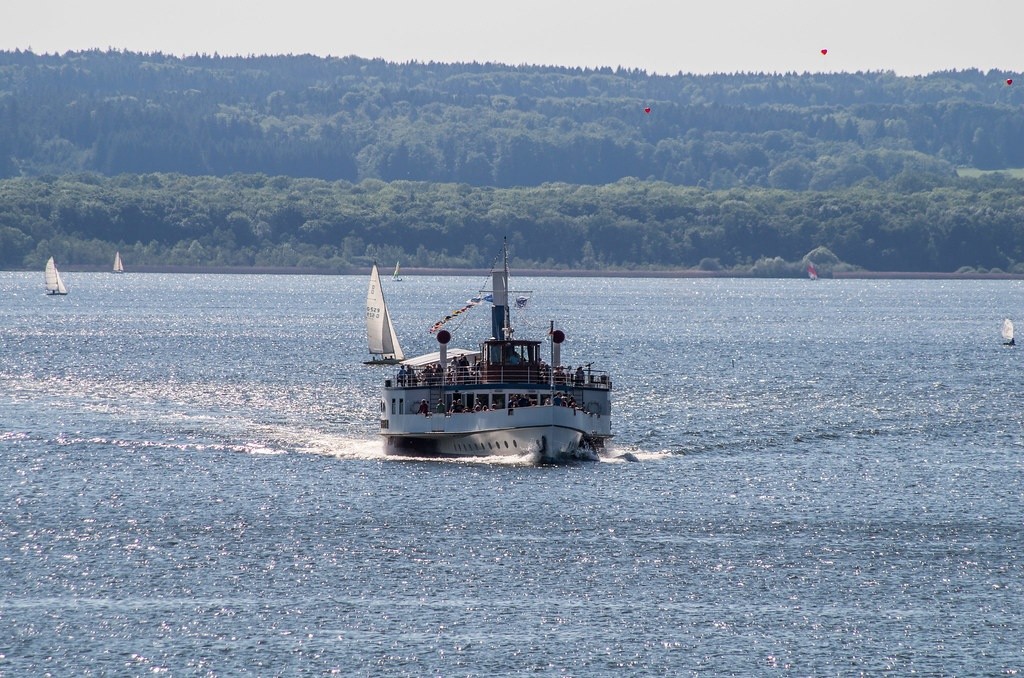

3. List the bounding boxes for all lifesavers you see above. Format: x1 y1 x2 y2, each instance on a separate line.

410 401 422 413
587 400 601 414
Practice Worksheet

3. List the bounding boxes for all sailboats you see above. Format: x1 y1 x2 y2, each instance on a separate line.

807 259 819 280
364 260 405 367
392 259 403 281
1002 318 1018 345
112 251 124 273
45 255 69 295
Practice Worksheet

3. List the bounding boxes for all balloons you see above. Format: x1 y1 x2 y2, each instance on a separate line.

821 50 827 55
645 107 650 113
1007 78 1012 85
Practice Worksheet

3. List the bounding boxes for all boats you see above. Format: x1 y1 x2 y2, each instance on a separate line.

375 236 613 469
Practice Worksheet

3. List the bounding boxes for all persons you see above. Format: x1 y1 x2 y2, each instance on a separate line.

416 399 429 417
510 352 526 368
451 353 470 384
397 365 407 386
492 399 497 411
553 366 566 384
407 365 414 386
538 358 548 384
473 398 487 412
544 392 575 410
436 399 446 414
450 398 464 413
421 363 443 384
575 367 584 386
508 394 531 408
